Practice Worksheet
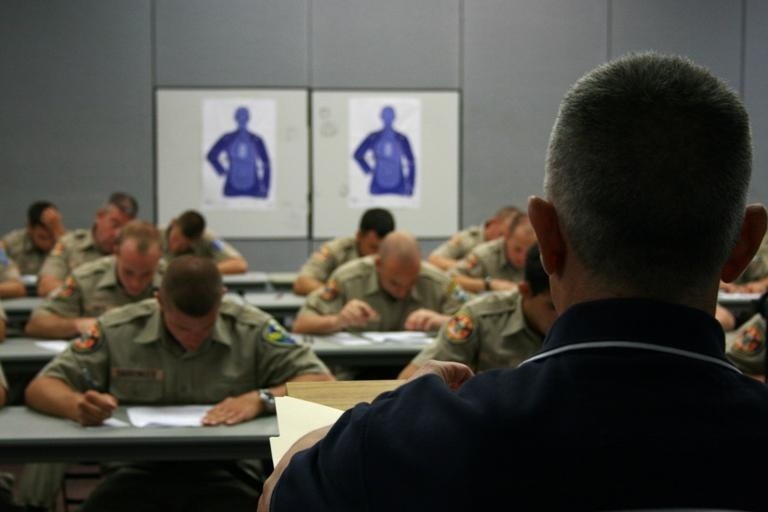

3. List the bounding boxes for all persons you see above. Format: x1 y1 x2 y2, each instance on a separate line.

256 50 767 511
292 205 559 389
710 235 768 370
1 192 339 512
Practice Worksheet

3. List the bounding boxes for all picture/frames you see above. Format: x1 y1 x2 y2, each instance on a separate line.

312 86 464 243
152 84 312 244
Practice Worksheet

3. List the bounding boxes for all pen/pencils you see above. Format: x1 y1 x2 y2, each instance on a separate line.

84 369 96 390
